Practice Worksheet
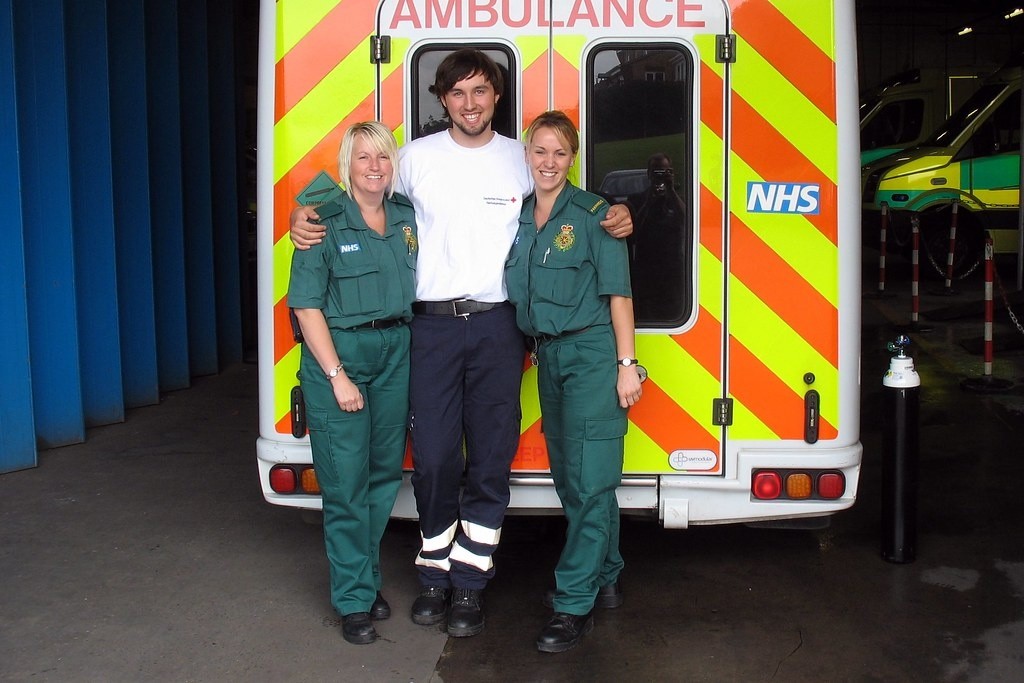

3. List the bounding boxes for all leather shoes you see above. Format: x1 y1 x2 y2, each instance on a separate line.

536 612 595 653
369 590 391 619
446 585 486 638
594 582 621 609
342 612 378 645
411 584 452 625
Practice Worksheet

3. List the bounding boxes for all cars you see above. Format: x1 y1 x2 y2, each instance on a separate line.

597 168 651 203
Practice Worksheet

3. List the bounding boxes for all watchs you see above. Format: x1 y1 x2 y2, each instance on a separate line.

616 358 638 366
325 362 343 380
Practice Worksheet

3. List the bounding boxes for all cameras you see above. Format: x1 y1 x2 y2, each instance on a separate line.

652 170 666 180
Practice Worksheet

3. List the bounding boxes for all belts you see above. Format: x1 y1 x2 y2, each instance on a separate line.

412 297 503 318
348 318 406 331
525 325 593 357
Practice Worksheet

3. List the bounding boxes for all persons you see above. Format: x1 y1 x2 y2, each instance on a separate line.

504 110 642 652
622 151 685 297
284 121 418 645
289 51 634 637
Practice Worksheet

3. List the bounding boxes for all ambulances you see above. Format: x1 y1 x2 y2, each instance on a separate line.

860 74 1023 275
257 0 863 529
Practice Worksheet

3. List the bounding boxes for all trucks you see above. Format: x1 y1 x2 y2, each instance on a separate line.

859 73 978 165
